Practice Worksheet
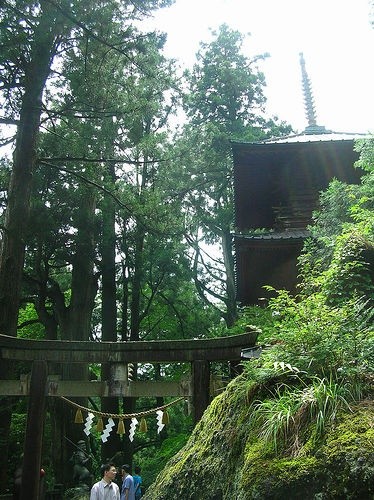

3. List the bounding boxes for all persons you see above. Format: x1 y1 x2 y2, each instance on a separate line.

134 466 142 500
88 464 120 500
119 464 135 500
